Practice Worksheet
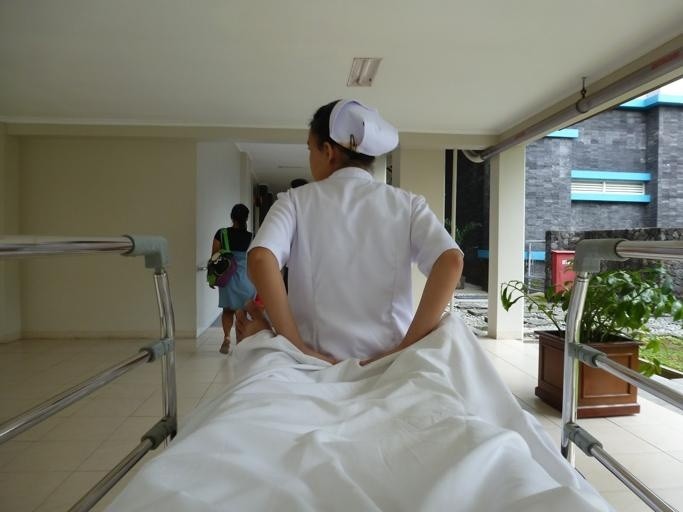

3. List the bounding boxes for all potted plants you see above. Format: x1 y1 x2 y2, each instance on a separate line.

498 263 683 419
444 217 484 289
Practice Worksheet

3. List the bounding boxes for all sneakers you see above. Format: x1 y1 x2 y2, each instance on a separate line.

220 337 231 354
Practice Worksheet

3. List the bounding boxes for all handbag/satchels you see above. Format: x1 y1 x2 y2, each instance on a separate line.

206 228 237 289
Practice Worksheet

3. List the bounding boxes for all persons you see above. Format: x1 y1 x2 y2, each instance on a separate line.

244 97 465 368
230 297 272 343
205 202 258 354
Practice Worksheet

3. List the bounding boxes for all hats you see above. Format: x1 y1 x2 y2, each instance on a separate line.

328 97 400 157
